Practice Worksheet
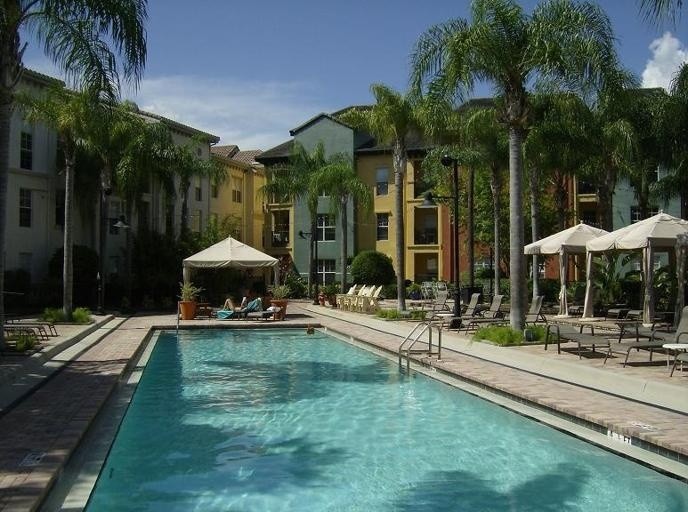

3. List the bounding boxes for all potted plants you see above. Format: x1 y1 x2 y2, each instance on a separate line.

177 281 202 320
266 284 292 319
321 284 341 307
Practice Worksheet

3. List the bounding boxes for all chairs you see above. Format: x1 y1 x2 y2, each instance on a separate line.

423 280 548 336
544 305 688 377
247 304 284 321
217 298 262 321
334 284 383 313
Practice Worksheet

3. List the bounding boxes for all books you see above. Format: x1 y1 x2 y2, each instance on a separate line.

242 296 248 306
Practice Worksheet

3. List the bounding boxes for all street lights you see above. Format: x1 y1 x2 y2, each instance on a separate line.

296 229 318 307
94 163 131 316
420 155 468 330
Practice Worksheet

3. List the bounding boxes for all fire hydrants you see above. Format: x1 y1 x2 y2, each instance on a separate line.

317 290 324 307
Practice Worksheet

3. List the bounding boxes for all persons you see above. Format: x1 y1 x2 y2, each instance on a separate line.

222 298 253 313
